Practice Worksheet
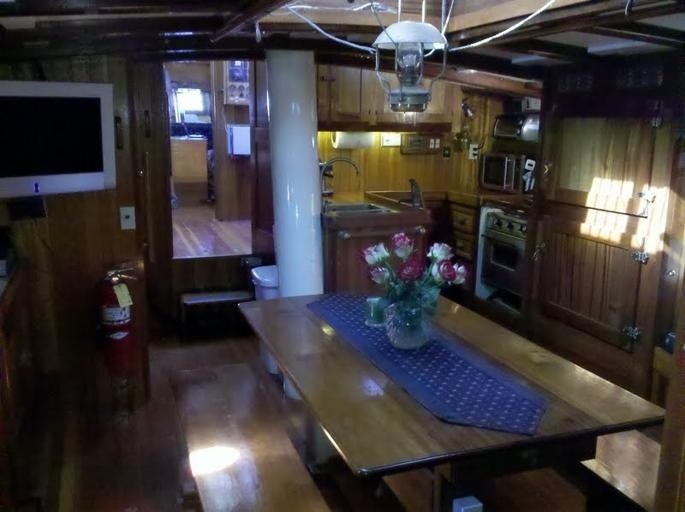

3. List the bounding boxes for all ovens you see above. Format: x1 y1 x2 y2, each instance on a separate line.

475 205 528 318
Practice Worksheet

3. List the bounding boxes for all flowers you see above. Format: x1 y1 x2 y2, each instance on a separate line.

359 231 472 331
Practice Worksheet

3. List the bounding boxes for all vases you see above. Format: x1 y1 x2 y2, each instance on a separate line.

381 301 434 353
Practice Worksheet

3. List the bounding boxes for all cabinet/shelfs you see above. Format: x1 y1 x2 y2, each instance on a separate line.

533 110 655 387
405 200 480 291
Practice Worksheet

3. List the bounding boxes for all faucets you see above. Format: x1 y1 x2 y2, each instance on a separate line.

320 155 360 181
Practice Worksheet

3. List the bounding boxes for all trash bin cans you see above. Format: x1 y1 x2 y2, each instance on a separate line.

251 264 280 375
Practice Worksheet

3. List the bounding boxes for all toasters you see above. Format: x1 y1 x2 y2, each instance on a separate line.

491 110 540 143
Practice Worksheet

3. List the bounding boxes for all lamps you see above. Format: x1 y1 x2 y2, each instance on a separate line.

285 2 554 114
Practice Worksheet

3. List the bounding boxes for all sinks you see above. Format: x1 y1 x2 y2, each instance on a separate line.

327 201 392 216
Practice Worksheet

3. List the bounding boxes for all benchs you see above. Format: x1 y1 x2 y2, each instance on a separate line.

166 361 333 511
580 428 664 512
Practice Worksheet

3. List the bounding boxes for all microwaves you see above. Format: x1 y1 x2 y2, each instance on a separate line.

479 152 536 195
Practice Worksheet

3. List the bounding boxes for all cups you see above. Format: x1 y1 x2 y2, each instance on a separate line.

365 296 387 328
664 331 681 354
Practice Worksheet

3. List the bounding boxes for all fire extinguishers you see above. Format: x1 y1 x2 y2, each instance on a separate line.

99 267 138 370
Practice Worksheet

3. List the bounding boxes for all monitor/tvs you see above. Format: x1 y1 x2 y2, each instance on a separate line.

0 81 117 196
